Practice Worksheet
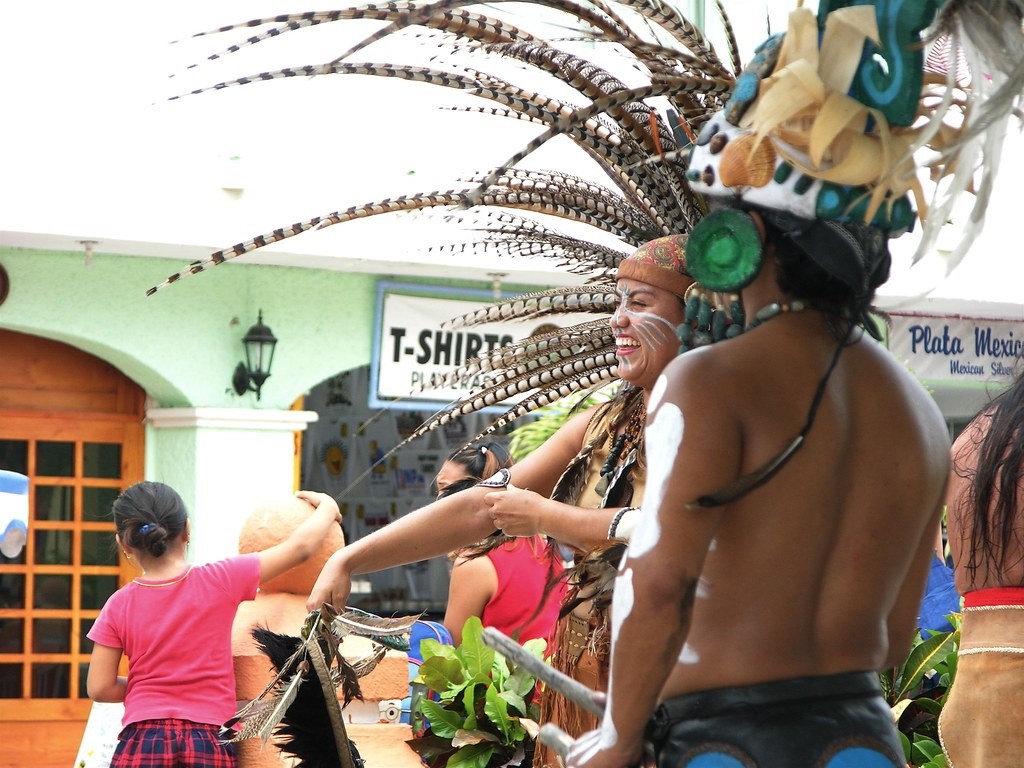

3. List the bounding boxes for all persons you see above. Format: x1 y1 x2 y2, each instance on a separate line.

85 481 342 767
306 231 717 768
939 369 1023 768
567 109 951 768
438 442 571 704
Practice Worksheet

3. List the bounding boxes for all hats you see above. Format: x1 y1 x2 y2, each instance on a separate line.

614 235 708 304
683 1 967 237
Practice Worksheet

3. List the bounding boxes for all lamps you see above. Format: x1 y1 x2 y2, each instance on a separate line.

232 308 277 401
